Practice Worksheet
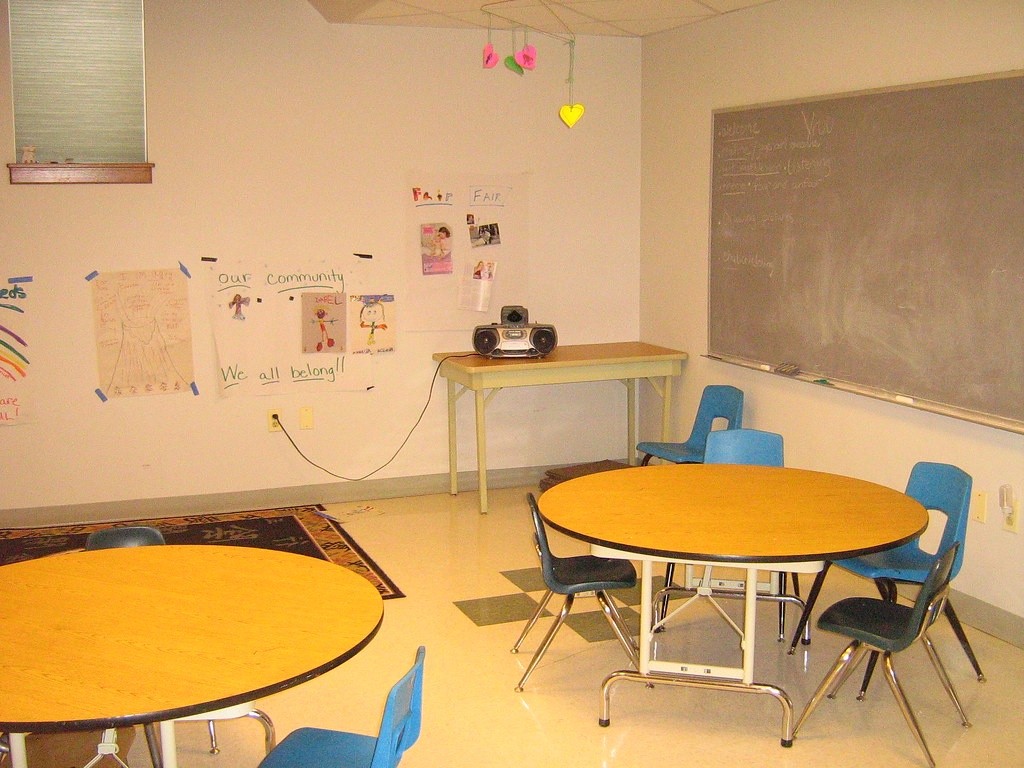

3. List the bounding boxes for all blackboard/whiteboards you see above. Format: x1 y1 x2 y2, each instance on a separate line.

701 68 1023 434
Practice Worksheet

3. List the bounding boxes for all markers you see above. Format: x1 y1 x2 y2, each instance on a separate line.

814 380 835 388
707 354 722 360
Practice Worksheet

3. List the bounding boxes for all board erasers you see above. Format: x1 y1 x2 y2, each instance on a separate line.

773 362 799 376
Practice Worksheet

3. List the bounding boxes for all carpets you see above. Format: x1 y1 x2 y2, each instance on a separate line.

0 503 407 601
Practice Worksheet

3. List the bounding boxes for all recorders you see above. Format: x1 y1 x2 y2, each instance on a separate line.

473 323 558 359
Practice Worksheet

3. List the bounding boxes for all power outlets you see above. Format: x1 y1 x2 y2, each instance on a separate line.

266 408 283 433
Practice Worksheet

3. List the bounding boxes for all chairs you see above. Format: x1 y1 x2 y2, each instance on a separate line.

256 645 426 768
792 540 974 768
650 428 801 644
83 526 222 755
510 491 654 693
635 384 744 467
787 461 987 702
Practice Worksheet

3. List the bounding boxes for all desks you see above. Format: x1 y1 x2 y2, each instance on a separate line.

537 463 930 748
432 341 688 515
0 544 385 768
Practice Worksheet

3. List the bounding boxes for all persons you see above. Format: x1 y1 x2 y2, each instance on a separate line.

466 215 473 223
432 227 452 257
473 261 494 280
479 227 492 244
